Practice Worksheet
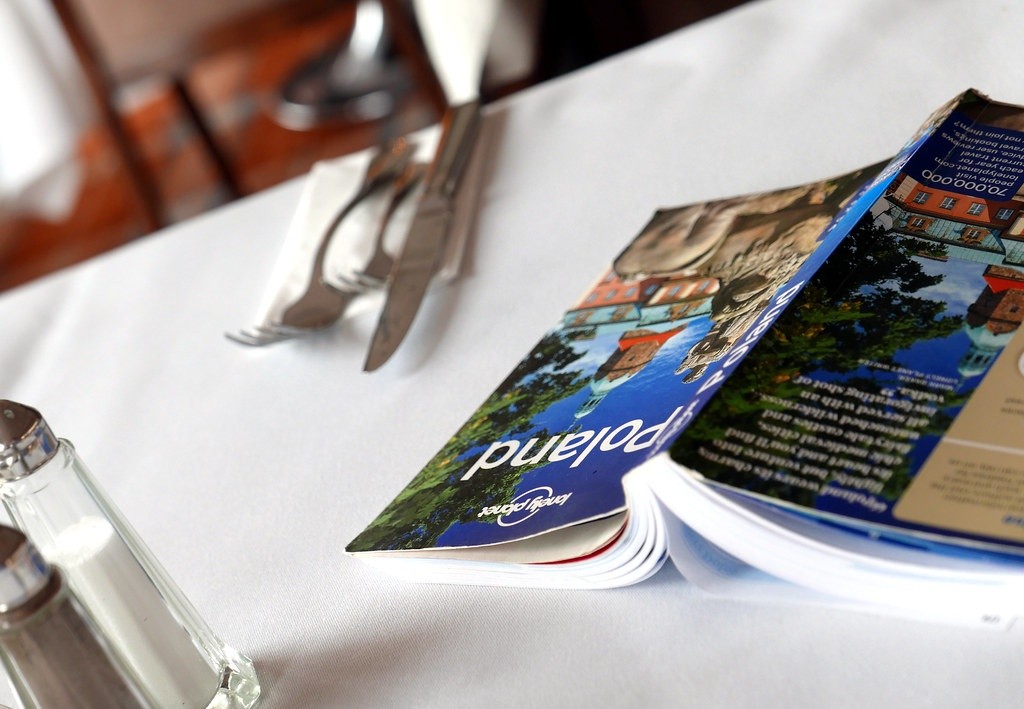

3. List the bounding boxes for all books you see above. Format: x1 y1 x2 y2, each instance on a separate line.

345 89 1024 629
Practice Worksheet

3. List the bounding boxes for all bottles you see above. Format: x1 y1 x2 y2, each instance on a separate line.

0 398 263 709
0 525 150 709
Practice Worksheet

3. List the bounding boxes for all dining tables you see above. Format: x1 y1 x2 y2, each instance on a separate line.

0 0 1024 709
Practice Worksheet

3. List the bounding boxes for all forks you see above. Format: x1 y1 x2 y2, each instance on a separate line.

225 136 408 343
317 163 422 297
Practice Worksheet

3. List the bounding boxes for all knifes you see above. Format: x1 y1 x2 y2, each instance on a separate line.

362 101 480 372
